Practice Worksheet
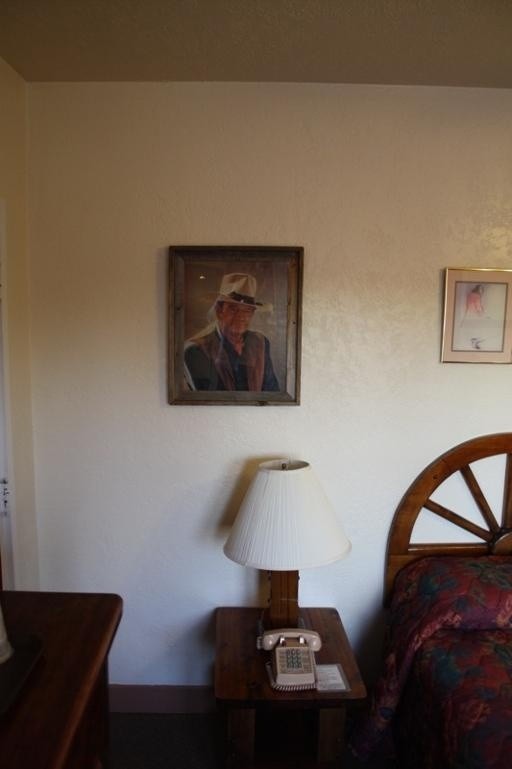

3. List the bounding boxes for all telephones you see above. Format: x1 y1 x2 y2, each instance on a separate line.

262 628 322 687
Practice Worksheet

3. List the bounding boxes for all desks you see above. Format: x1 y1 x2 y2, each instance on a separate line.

0 591 123 769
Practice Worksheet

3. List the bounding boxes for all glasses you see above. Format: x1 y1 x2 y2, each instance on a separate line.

226 305 254 317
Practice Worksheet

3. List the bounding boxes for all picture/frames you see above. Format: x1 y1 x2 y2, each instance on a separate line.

441 267 512 364
168 246 304 406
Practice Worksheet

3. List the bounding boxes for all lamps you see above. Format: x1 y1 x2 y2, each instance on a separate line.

224 458 353 626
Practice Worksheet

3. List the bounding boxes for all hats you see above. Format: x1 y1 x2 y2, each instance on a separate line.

215 273 262 310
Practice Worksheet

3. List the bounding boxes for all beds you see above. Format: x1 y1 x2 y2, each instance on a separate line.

383 431 512 769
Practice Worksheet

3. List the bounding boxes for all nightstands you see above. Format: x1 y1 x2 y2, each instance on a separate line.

216 608 366 769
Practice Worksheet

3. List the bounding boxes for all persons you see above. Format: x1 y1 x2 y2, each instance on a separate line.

458 283 490 351
180 269 280 394
